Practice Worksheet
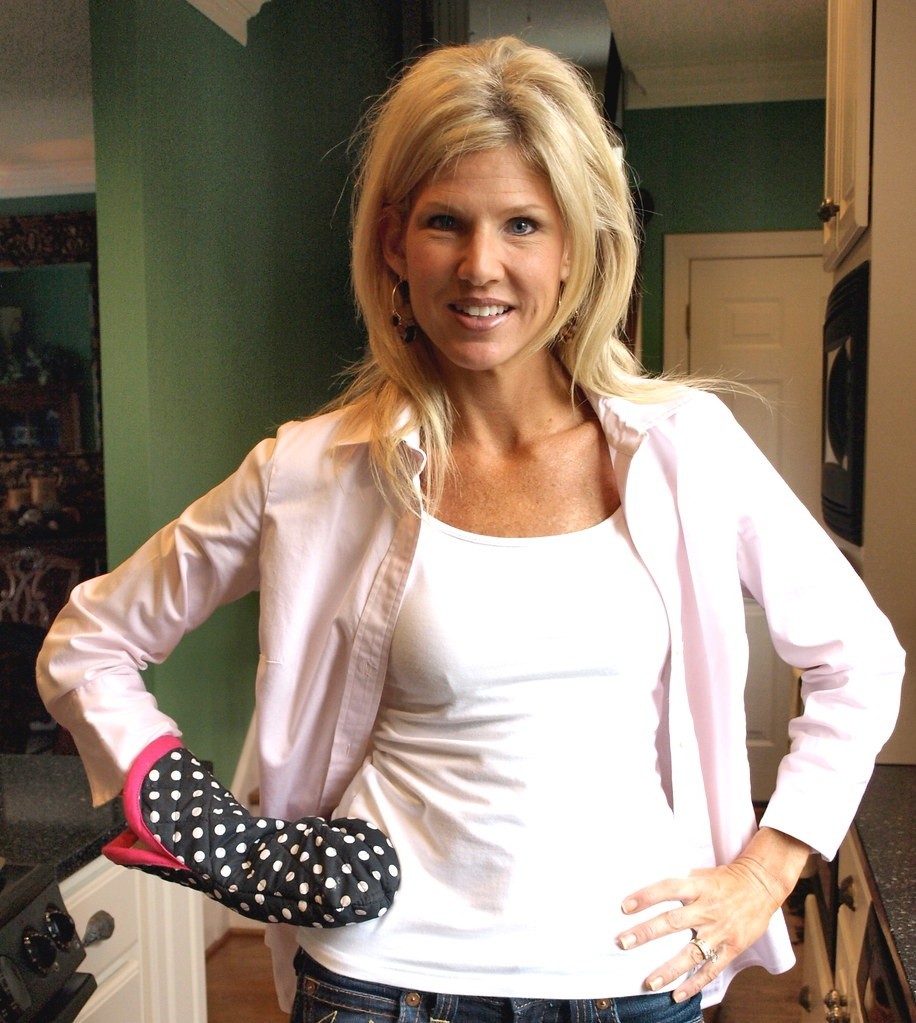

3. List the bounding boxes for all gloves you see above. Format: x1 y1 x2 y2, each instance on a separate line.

102 733 402 929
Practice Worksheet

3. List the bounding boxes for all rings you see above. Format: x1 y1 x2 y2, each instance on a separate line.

689 939 715 960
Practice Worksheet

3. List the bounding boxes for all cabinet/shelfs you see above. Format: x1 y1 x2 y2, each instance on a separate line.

817 1 916 271
58 853 206 1023
796 829 906 1023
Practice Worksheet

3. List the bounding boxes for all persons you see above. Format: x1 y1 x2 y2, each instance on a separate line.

35 35 908 1023
